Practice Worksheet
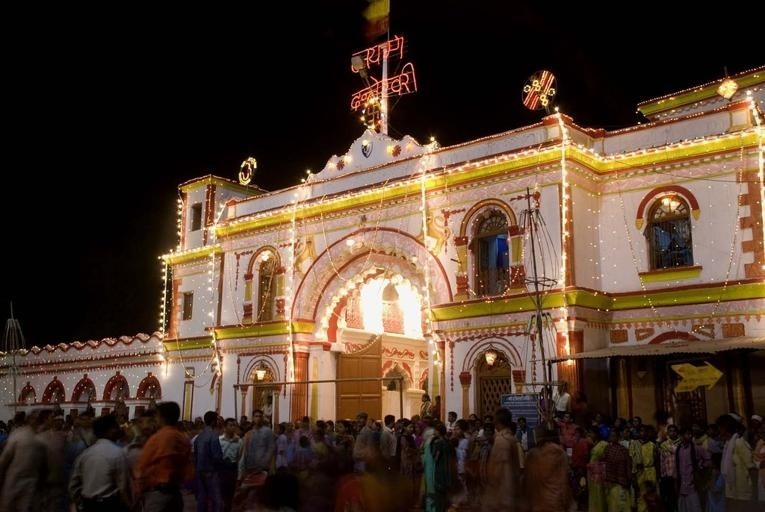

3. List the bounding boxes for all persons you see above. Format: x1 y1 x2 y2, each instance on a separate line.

536 387 552 422
553 385 571 419
572 391 587 419
433 395 440 421
1 401 765 512
419 394 432 417
260 395 272 423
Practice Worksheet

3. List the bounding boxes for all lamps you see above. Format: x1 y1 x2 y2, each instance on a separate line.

256 360 266 380
484 343 497 366
